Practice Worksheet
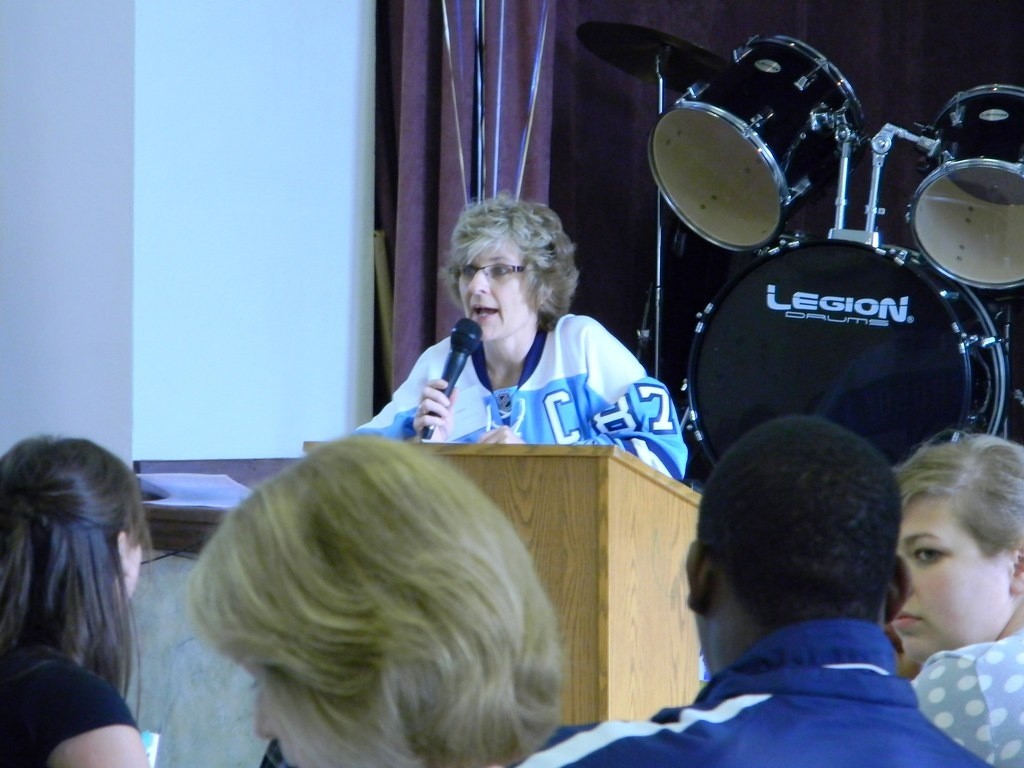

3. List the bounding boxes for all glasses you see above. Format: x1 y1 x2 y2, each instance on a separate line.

449 263 525 285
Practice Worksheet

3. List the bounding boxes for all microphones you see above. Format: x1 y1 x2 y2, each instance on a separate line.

420 318 483 439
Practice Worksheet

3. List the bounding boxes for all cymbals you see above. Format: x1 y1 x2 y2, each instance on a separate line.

573 18 733 94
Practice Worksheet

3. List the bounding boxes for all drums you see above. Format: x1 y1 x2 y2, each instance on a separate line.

646 31 868 255
908 82 1024 290
687 238 1010 472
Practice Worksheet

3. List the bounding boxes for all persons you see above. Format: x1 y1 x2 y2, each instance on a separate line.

500 413 995 768
190 434 562 768
892 434 1024 768
355 194 688 480
0 435 153 765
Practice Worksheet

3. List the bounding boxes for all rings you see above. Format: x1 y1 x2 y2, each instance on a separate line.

419 403 427 414
